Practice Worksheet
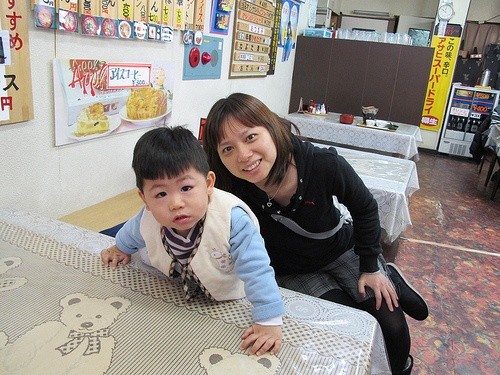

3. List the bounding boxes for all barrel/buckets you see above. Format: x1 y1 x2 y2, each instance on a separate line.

407 28 430 46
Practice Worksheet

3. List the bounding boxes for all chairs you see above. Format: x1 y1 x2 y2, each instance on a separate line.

478 113 491 174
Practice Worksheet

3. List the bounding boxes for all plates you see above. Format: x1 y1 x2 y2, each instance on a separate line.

194 31 202 45
101 18 116 37
38 8 53 28
68 116 121 141
61 12 77 32
118 21 132 38
135 23 146 39
183 32 193 44
82 16 98 35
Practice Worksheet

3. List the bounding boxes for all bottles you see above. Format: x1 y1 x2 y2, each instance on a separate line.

315 104 321 115
311 104 317 114
321 104 326 114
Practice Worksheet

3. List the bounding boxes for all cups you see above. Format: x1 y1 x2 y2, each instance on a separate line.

366 119 375 126
388 124 399 130
337 27 412 46
354 117 363 125
339 115 353 124
375 120 387 128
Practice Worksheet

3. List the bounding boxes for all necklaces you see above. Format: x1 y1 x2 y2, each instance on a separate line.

266 167 289 207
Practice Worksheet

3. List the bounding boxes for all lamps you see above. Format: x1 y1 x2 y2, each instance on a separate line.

354 10 390 17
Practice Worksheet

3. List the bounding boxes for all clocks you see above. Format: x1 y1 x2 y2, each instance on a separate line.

438 5 454 19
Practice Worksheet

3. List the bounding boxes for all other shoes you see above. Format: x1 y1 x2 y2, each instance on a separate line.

386 262 429 321
401 354 413 375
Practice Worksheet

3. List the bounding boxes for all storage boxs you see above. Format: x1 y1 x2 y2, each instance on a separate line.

303 29 332 38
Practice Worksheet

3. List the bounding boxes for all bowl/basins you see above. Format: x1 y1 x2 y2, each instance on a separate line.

119 99 172 126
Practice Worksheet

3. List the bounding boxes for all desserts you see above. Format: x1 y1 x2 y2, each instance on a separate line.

73 102 110 136
126 87 167 120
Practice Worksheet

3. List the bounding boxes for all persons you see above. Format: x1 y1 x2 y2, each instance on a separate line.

203 93 430 375
100 124 286 356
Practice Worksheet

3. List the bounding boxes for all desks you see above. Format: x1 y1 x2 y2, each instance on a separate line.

0 209 392 375
484 124 500 187
302 140 420 263
285 111 423 162
